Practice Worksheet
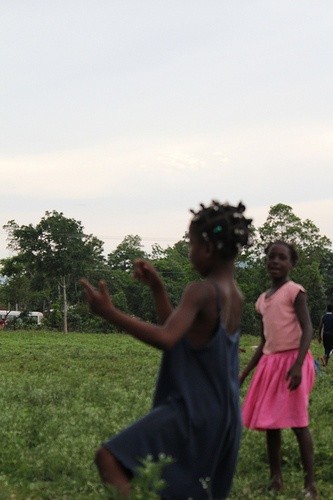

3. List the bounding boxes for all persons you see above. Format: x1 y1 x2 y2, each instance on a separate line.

239 240 321 500
79 200 252 500
318 305 333 367
